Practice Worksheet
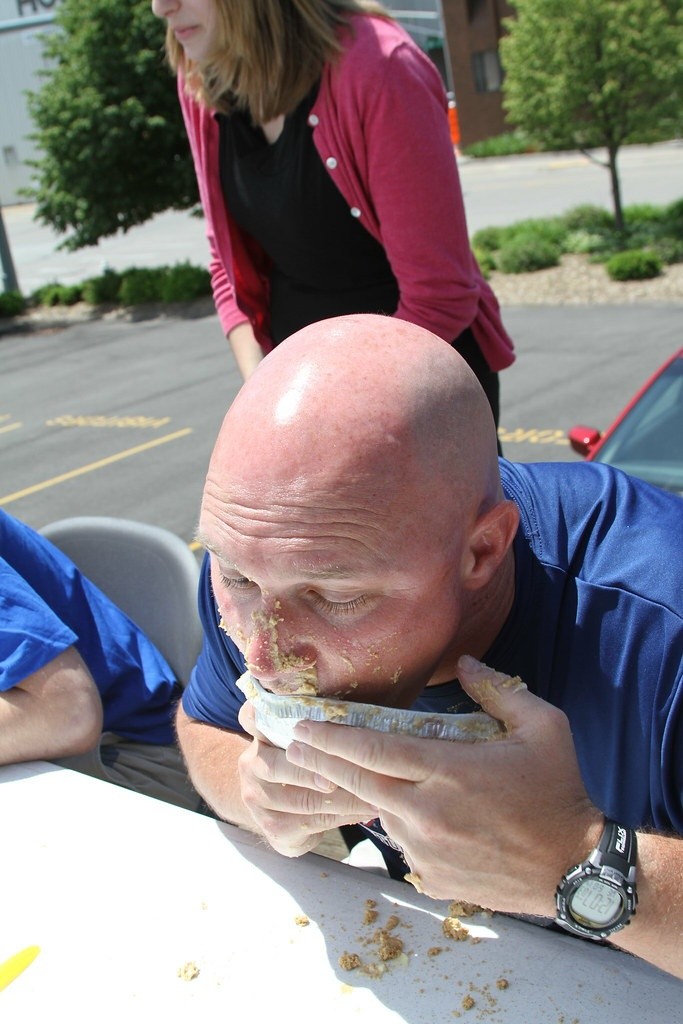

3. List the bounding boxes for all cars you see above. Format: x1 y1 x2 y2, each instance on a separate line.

564 344 682 514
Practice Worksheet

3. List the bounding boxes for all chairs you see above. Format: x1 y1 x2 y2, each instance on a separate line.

34 515 203 687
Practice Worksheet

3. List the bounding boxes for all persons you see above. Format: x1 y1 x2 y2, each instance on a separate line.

153 0 516 458
174 311 682 984
1 507 199 813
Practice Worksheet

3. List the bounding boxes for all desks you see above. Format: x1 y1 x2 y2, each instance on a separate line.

0 760 683 1024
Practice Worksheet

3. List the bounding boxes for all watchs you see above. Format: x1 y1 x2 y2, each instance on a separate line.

552 818 639 943
